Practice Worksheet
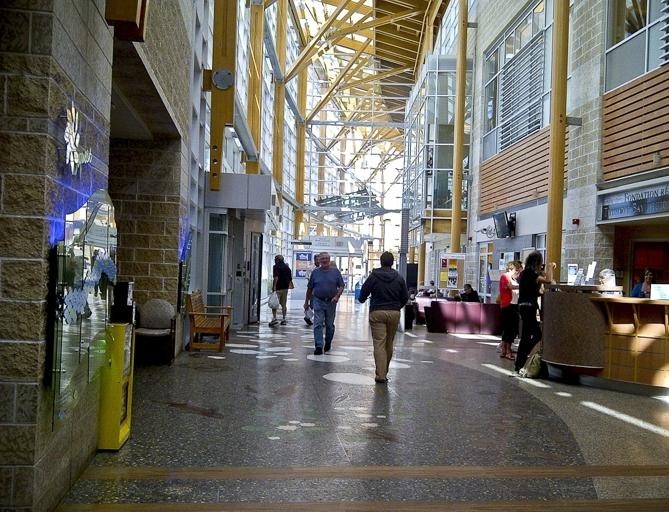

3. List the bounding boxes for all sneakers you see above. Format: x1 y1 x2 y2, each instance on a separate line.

269 320 287 326
375 375 387 382
314 341 331 354
304 317 314 325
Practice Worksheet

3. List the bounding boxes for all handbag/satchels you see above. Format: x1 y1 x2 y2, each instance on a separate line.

519 352 541 378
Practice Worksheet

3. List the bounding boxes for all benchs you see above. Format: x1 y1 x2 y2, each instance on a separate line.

186 290 234 354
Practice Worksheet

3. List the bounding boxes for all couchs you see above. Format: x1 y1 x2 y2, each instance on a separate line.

414 296 506 335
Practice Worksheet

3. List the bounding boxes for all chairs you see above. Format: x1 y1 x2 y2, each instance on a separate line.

131 299 175 371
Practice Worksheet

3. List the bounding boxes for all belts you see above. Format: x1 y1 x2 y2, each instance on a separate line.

316 296 332 302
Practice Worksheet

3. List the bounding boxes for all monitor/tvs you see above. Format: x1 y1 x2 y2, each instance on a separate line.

493 210 513 238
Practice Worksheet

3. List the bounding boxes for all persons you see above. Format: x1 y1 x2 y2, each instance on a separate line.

498 261 519 360
302 252 345 356
495 262 524 352
357 251 408 382
302 253 322 326
630 269 655 298
510 252 554 379
267 255 291 327
598 269 626 297
459 283 480 303
